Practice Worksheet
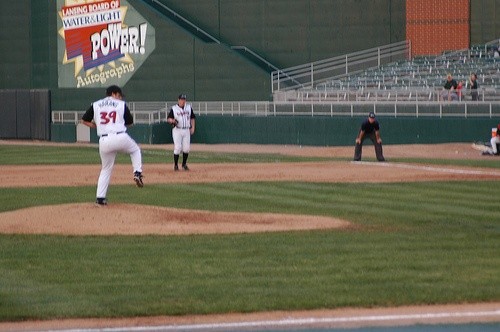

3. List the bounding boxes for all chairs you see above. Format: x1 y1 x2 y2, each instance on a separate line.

298 43 500 99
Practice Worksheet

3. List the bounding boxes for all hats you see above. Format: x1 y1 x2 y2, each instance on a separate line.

178 94 187 100
106 85 124 100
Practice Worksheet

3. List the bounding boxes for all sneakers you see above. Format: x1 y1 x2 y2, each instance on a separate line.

369 112 375 118
133 176 143 187
96 198 108 206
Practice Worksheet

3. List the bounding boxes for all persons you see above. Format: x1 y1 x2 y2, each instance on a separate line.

167 93 196 172
353 113 386 161
439 73 481 102
81 85 144 205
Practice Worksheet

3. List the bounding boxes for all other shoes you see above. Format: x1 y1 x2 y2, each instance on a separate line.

182 165 188 170
174 166 179 171
